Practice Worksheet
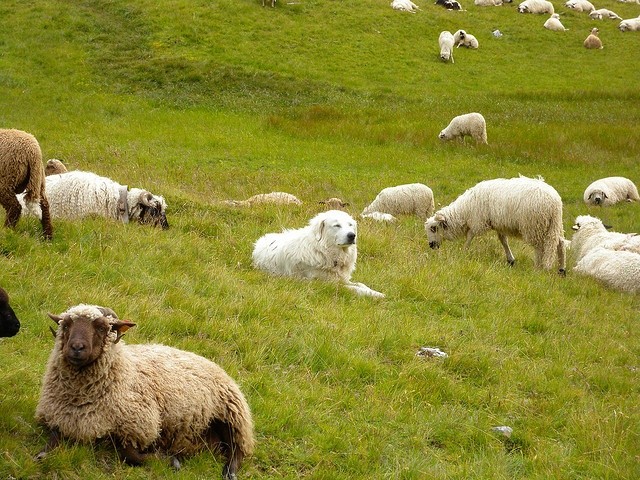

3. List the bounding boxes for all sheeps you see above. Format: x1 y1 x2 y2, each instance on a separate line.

390 0 419 12
425 172 566 277
584 28 603 49
439 31 455 64
589 9 623 21
438 112 488 144
360 183 435 222
15 169 169 230
544 13 569 31
619 15 640 32
45 159 67 175
0 285 20 338
437 0 462 9
572 0 595 12
566 0 573 8
474 0 512 7
0 128 53 240
453 29 479 48
34 303 254 479
319 197 350 210
518 0 554 15
584 177 640 205
571 214 640 294
224 192 301 207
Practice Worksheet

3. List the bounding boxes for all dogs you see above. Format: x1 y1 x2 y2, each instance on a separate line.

250 210 386 299
